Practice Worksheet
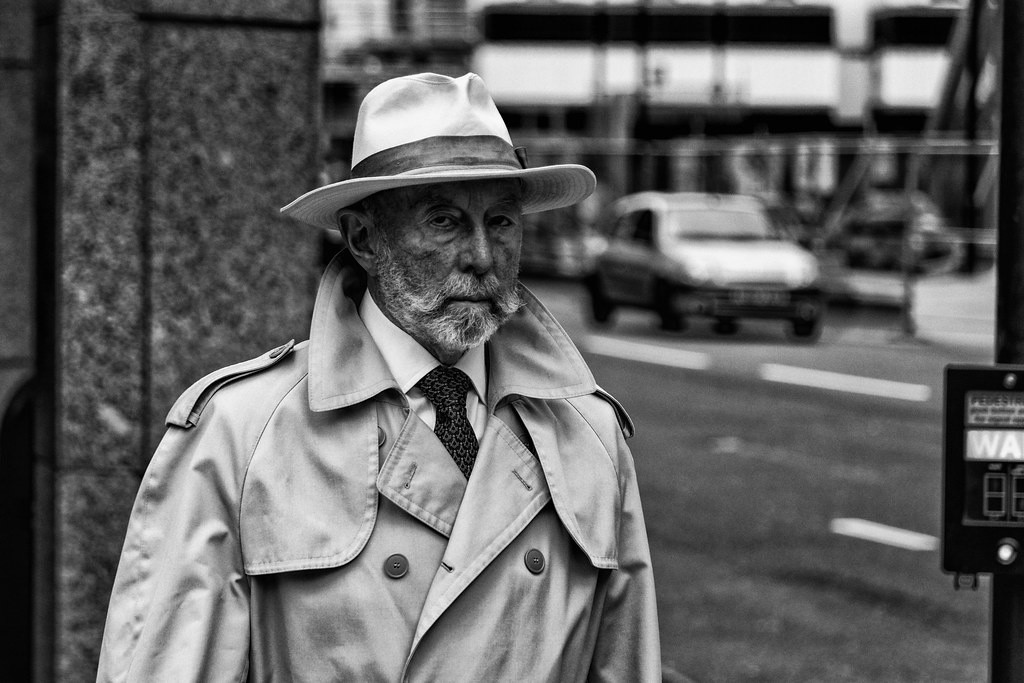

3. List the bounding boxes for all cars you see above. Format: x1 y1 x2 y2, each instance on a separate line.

841 192 961 277
587 188 829 341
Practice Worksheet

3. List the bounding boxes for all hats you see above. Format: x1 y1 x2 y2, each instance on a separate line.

280 72 596 230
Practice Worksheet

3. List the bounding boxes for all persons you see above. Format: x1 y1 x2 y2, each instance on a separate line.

94 71 663 683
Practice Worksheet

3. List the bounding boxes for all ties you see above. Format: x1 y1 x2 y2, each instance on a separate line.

413 365 479 482
